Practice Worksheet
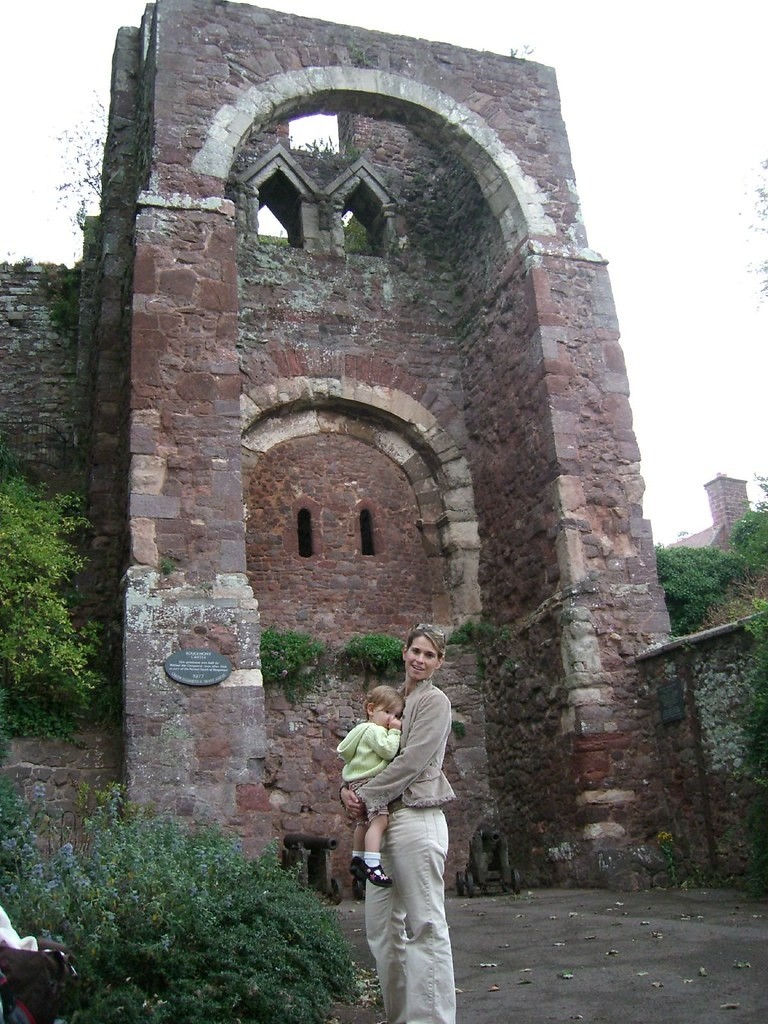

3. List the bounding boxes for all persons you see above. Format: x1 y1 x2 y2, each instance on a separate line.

337 687 405 887
339 624 458 1024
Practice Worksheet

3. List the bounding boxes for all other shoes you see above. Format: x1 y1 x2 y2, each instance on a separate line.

350 857 392 887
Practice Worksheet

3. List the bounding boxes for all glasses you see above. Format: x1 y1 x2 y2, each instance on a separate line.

410 623 446 654
